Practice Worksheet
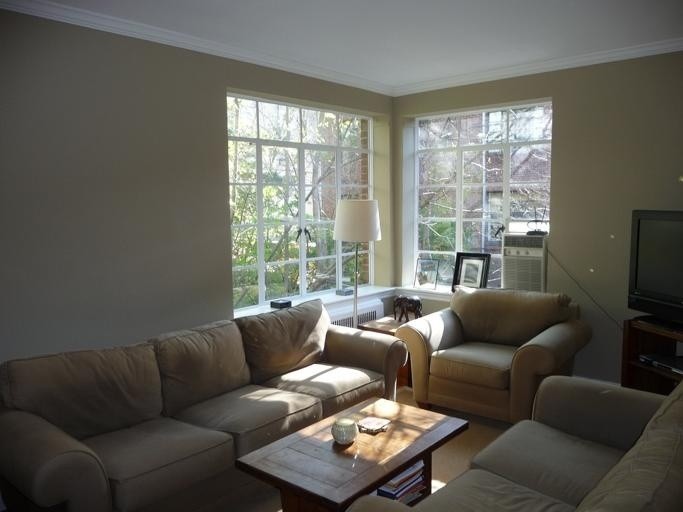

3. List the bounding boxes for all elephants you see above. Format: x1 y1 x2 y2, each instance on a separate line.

392 295 423 323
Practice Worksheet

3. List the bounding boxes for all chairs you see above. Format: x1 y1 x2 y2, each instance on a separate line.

393 286 593 424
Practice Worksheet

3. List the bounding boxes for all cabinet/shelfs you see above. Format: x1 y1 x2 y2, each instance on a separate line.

620 316 683 393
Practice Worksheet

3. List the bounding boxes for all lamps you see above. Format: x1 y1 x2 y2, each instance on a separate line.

332 197 382 329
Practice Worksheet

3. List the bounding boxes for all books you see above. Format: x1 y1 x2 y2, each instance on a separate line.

379 458 426 504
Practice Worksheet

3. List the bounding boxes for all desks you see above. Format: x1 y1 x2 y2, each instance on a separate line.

356 314 428 388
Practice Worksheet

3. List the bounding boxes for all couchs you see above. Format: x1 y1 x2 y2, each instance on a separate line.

341 374 683 512
0 298 410 512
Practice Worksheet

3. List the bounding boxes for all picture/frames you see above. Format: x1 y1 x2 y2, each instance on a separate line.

451 250 491 292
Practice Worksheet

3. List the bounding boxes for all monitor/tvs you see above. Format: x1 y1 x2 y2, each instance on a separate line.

628 209 683 334
